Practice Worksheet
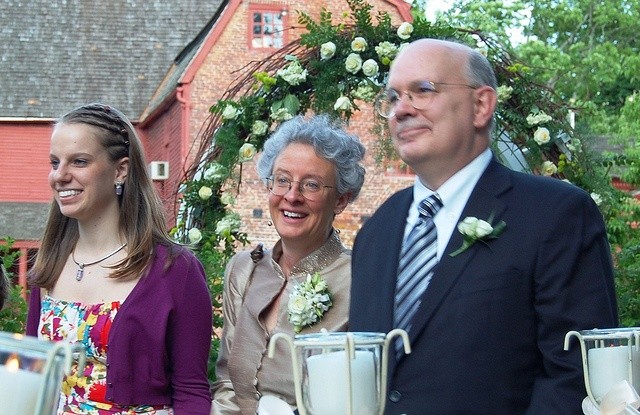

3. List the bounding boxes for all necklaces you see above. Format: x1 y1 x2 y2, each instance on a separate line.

69 240 126 282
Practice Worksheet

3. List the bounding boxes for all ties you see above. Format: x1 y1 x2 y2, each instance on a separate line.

392 193 444 366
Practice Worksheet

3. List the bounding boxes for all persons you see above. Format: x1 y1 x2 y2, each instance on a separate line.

24 102 212 415
207 111 365 414
345 36 618 415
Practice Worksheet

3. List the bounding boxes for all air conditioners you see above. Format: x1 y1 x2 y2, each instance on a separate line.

147 160 169 181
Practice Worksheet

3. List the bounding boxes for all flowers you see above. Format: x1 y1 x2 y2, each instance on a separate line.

334 95 352 113
375 41 409 65
346 53 361 73
287 272 331 332
204 159 226 183
223 105 237 119
252 119 266 135
276 59 307 87
240 143 255 161
269 104 291 120
539 160 557 177
497 84 512 104
449 209 506 258
534 126 551 146
350 36 367 51
474 35 487 57
363 58 378 76
188 227 202 242
220 192 233 206
320 41 337 58
198 186 211 198
397 21 414 40
217 214 247 241
565 110 575 128
527 109 552 125
590 192 602 207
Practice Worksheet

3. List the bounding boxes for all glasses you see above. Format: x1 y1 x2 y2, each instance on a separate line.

265 174 337 201
374 79 476 119
